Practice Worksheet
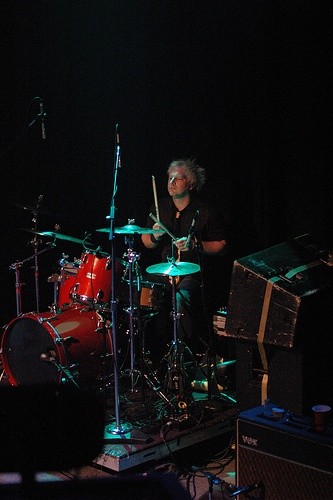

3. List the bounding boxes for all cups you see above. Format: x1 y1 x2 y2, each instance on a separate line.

312 405 331 431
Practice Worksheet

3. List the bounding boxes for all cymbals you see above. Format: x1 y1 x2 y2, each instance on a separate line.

95 224 167 234
25 228 93 245
146 261 201 275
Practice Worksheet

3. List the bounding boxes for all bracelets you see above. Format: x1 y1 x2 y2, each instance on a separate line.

150 233 160 243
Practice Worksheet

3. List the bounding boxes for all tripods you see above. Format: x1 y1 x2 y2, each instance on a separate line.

98 234 221 420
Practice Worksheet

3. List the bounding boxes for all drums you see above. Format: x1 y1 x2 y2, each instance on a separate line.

1 309 113 389
57 261 78 308
69 248 129 312
126 279 166 312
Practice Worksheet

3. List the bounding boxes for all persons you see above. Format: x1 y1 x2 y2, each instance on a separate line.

136 158 227 392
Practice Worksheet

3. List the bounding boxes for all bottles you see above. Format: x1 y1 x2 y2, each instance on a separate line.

191 380 227 393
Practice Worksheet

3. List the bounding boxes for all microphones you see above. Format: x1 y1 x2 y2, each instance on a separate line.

185 211 199 247
229 483 259 497
116 125 122 164
40 350 56 362
40 99 47 140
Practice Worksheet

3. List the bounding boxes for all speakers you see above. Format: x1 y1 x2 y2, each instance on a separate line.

224 233 333 500
0 470 192 500
